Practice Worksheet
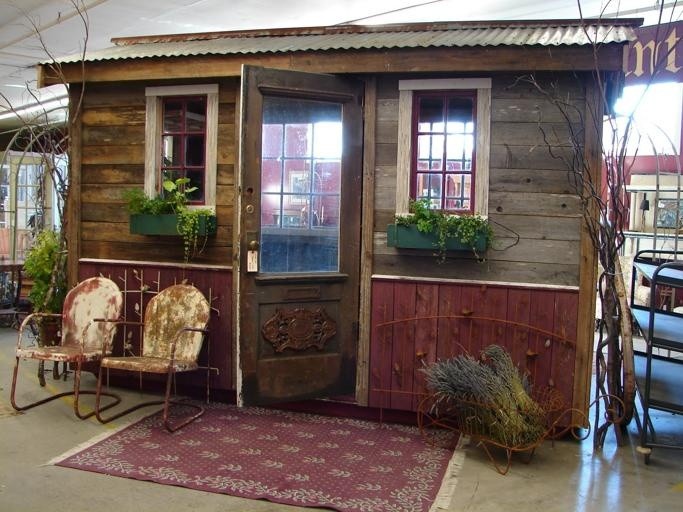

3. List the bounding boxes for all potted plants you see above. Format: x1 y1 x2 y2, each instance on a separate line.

19 224 69 348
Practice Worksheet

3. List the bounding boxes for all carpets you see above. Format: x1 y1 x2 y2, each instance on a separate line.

41 395 472 512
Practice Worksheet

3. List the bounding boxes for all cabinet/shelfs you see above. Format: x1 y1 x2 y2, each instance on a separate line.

629 249 683 463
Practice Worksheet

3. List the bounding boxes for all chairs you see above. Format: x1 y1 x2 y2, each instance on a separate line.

9 275 213 434
